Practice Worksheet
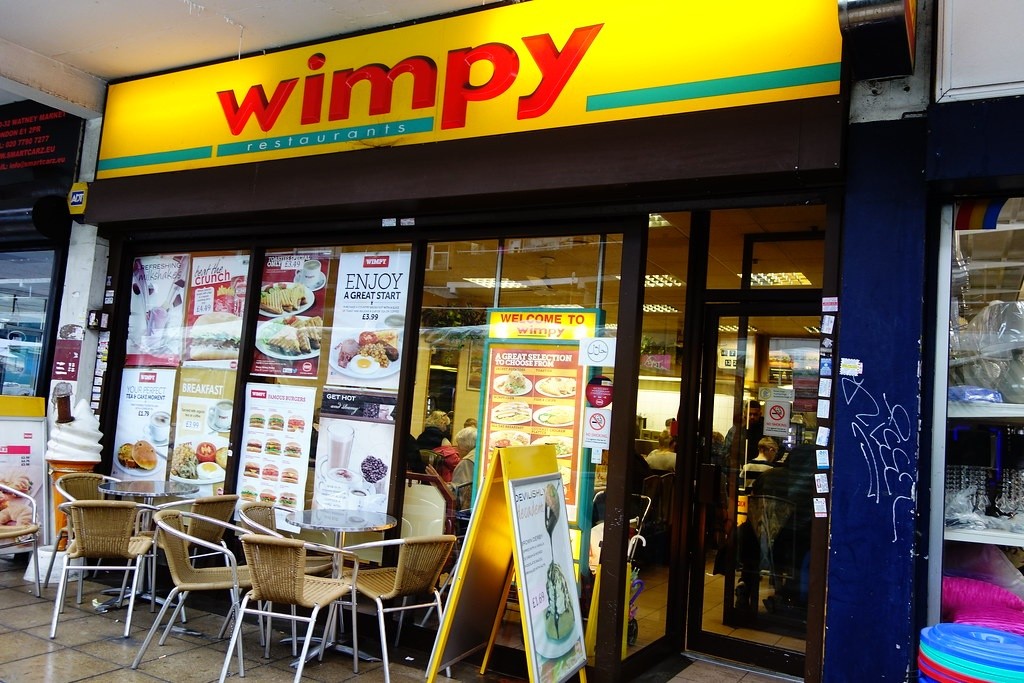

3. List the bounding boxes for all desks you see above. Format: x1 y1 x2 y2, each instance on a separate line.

280 510 399 670
96 481 199 614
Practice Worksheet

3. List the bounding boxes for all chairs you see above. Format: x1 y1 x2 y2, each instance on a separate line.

218 534 361 683
43 473 152 607
50 500 162 646
117 493 241 637
629 472 674 570
399 471 472 572
132 509 266 678
318 535 457 683
737 494 810 627
238 502 347 660
0 483 42 598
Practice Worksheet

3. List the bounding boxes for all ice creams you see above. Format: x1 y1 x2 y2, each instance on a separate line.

44 398 104 548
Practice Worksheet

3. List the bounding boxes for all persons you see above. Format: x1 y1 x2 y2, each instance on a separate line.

404 410 480 513
632 400 819 612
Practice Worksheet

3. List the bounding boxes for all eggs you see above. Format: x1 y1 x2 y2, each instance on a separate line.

197 462 225 480
349 355 380 374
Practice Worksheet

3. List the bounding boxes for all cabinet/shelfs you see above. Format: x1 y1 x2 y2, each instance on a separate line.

0 278 51 397
926 198 1024 627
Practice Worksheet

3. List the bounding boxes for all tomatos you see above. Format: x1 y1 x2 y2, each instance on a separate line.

196 442 217 461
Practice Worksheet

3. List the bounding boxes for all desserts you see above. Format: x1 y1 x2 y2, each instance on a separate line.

361 455 388 496
543 564 575 640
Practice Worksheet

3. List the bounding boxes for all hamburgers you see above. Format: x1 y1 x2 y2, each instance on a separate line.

189 313 243 359
117 440 157 470
241 412 305 507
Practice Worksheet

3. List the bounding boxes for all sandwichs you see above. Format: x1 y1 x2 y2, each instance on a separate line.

267 315 322 355
260 284 306 313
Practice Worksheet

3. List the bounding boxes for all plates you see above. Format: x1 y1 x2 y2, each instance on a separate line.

530 436 573 458
533 611 579 658
294 272 326 291
494 375 532 395
114 454 162 476
170 471 225 485
491 408 532 424
533 405 574 426
4 472 42 523
256 315 320 360
489 430 530 450
208 418 230 432
144 425 169 448
259 282 315 317
535 377 576 398
329 341 402 379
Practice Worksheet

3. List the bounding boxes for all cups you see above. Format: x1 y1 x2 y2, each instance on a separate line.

143 411 171 441
327 423 354 469
296 260 321 284
209 399 233 429
